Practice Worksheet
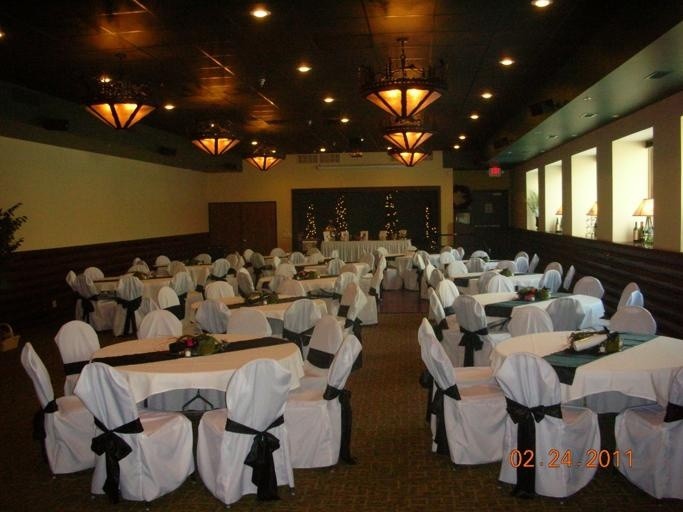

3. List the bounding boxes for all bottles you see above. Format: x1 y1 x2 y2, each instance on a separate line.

555 218 560 232
632 221 646 240
573 328 615 351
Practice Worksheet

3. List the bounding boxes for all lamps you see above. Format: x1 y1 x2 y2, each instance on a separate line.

586 202 598 237
632 198 653 243
556 206 562 231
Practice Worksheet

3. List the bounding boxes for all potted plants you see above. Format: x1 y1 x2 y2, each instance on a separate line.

527 191 538 228
